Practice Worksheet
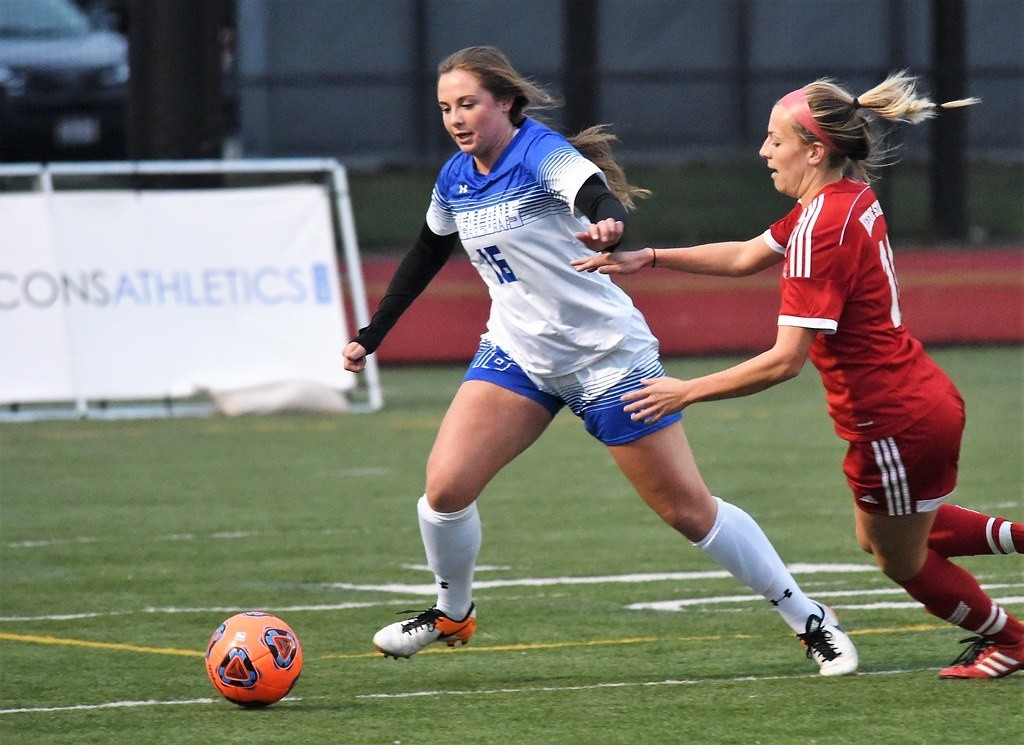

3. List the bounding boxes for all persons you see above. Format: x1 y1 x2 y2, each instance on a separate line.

77 0 241 188
340 45 861 676
571 67 1024 680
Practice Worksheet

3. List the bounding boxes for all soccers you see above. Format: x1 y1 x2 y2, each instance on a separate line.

203 608 305 710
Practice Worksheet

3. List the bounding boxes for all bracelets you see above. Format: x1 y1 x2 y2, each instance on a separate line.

650 247 656 268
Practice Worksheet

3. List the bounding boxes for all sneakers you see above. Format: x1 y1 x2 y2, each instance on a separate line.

796 599 859 676
939 635 1024 679
372 600 476 660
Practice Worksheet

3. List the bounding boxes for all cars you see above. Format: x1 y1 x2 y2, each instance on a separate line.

0 0 131 112
